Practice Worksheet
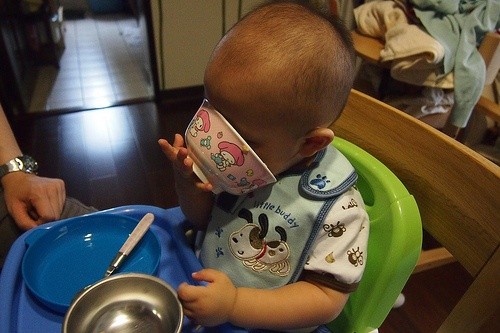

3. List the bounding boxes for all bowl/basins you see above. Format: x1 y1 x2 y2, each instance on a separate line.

183 97 278 197
60 273 183 333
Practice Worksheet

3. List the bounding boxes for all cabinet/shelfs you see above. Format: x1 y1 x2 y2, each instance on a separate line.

16 0 66 73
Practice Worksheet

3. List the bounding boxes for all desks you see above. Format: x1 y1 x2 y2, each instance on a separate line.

0 204 332 333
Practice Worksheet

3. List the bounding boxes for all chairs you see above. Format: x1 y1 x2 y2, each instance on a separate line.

325 136 424 333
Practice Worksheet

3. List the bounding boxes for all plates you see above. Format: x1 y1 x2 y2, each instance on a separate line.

19 213 164 312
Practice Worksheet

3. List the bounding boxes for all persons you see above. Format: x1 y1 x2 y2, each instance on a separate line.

0 104 99 274
157 0 371 333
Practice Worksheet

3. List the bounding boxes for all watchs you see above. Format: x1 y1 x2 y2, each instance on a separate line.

0 155 38 179
